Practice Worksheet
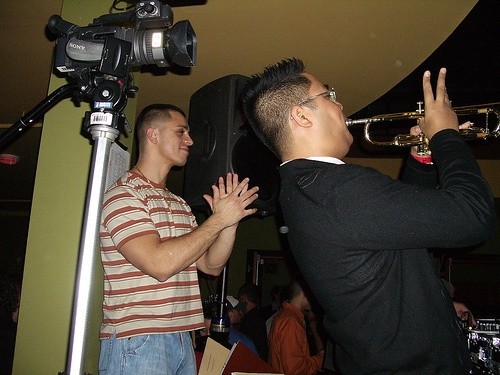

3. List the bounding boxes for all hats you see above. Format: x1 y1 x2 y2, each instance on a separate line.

216 296 249 316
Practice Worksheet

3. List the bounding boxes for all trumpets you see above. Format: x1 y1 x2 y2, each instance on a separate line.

345 102 500 150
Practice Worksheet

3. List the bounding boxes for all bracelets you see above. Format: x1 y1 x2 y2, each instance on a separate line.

411 154 434 165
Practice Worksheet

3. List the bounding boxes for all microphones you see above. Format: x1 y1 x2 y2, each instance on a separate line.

158 0 207 8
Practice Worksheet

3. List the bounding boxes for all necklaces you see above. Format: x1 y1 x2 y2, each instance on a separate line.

134 166 173 215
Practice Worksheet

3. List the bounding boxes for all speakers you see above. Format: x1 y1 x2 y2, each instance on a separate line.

185 74 281 217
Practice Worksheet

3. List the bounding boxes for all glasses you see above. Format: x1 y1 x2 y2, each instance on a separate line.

290 88 336 120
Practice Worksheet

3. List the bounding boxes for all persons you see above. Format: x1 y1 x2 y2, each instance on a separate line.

200 303 228 347
240 57 497 375
237 284 267 363
267 279 325 375
98 103 260 375
212 297 259 358
